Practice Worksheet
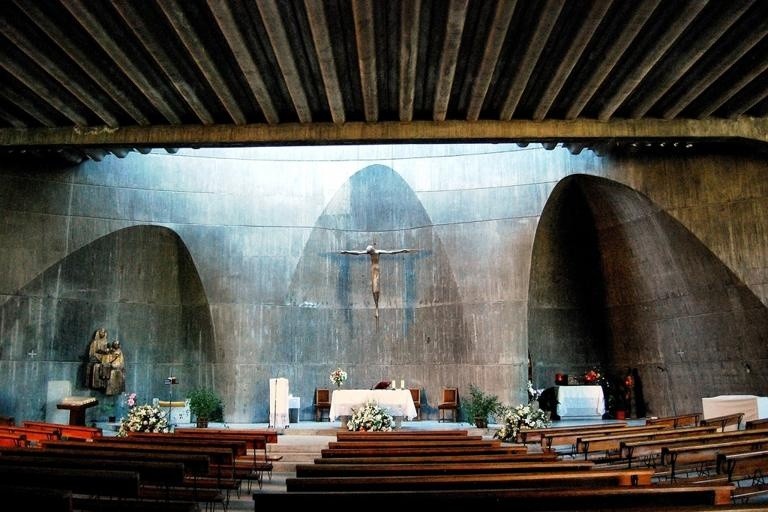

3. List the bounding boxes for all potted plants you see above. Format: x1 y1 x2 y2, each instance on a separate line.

186 385 224 428
463 384 503 428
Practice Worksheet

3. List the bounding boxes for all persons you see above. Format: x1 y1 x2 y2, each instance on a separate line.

103 339 126 394
81 328 110 387
336 244 421 322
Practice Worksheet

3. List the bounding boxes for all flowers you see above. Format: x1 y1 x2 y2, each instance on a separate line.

116 404 169 438
494 380 553 444
346 401 396 433
329 367 348 386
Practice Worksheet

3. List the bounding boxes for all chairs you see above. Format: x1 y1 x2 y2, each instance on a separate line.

315 386 458 424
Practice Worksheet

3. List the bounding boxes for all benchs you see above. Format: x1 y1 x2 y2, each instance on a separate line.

0 411 768 512
